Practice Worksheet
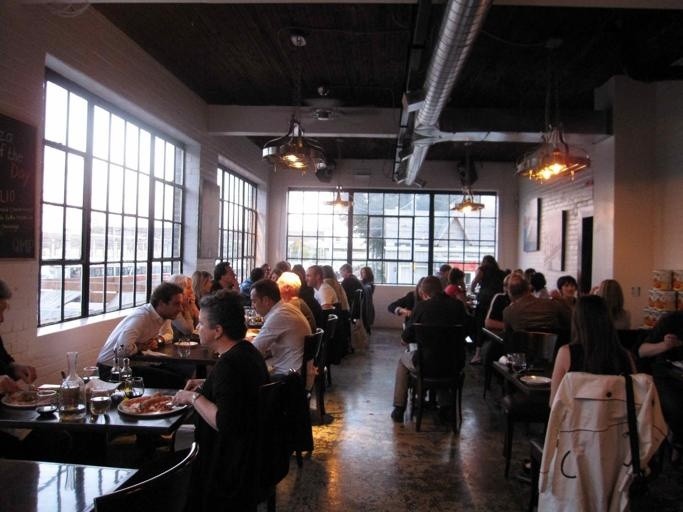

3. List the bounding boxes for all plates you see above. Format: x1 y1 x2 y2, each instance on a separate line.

519 375 552 386
1 391 53 408
173 341 198 347
117 395 189 417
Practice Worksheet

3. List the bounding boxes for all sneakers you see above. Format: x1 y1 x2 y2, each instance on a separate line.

515 462 531 483
391 406 404 422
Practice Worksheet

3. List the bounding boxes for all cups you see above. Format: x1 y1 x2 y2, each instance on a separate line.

36 365 145 417
512 352 527 375
506 354 513 369
247 311 256 326
177 336 191 359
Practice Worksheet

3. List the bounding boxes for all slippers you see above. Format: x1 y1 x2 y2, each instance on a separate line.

468 358 482 365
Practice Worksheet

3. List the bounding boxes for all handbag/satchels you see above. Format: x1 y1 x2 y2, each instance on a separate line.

629 462 681 511
350 319 370 350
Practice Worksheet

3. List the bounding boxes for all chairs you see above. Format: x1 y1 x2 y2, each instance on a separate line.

401 323 468 433
617 327 674 405
529 371 670 512
514 329 559 370
93 442 200 512
227 284 375 511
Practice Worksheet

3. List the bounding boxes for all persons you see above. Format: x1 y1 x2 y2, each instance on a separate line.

389 265 476 416
96 261 373 389
0 279 39 404
470 256 682 416
120 289 272 490
514 294 639 482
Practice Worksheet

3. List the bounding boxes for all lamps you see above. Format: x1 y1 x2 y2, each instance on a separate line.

262 78 337 177
322 184 354 207
451 184 484 212
512 63 590 184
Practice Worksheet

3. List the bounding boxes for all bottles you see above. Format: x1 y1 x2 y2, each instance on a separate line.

59 351 132 421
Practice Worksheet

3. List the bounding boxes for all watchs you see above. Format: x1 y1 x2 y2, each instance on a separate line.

191 392 200 405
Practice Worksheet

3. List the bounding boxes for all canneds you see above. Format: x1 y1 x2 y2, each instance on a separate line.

643 270 683 328
